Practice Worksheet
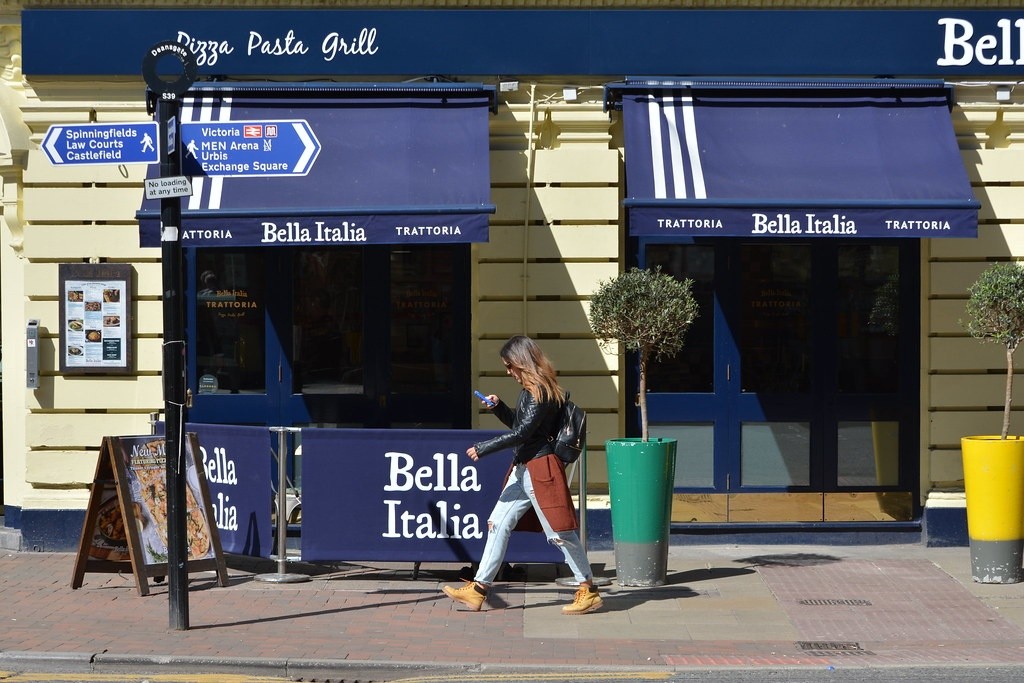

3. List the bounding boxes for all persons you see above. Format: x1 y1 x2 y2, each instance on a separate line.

440 333 605 616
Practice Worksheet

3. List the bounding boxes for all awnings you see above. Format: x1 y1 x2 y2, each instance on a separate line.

133 78 495 242
602 75 982 239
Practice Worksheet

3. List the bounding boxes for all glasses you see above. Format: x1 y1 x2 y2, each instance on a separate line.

504 362 512 369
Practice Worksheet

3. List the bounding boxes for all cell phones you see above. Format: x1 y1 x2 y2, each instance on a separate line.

474 390 495 407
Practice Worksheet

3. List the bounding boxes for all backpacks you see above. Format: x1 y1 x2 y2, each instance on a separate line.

547 390 587 463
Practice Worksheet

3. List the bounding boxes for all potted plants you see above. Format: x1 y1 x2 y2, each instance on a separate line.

955 261 1024 585
863 272 901 485
589 263 701 589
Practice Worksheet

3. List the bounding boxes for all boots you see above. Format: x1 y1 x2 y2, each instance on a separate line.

562 585 603 615
441 578 487 612
